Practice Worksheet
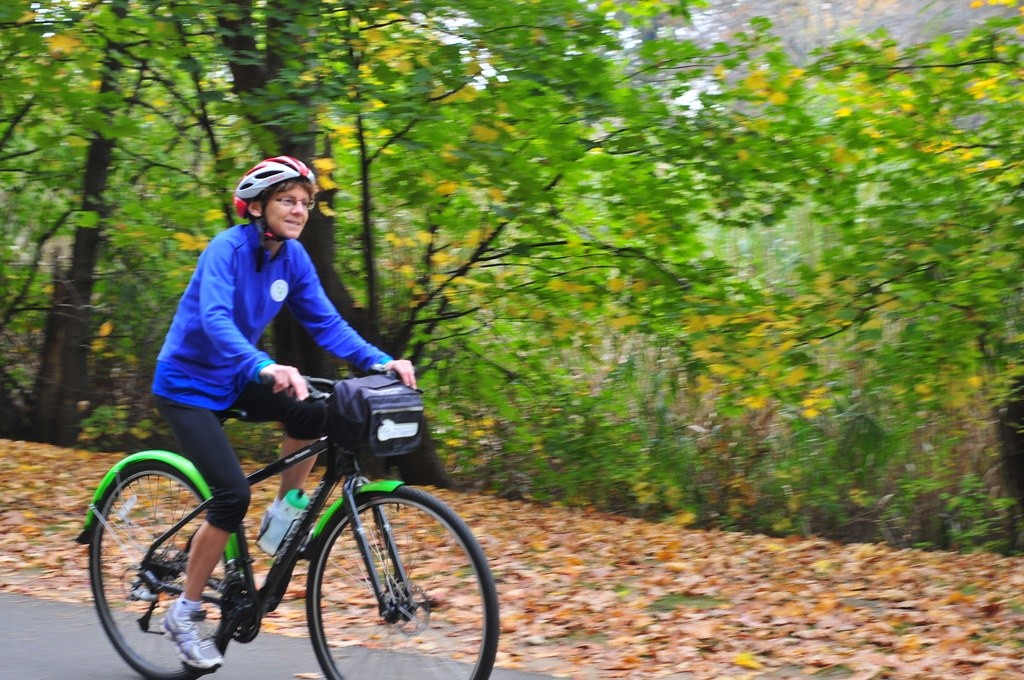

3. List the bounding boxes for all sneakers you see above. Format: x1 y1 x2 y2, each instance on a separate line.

255 507 273 537
160 597 224 672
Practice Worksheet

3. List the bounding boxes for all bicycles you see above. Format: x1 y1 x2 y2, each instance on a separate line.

74 377 501 680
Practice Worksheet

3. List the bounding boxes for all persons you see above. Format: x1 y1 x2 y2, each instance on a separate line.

151 156 418 669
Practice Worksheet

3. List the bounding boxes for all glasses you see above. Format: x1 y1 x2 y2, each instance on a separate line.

261 195 313 214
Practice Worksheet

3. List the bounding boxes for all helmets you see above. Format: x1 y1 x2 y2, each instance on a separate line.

233 155 318 219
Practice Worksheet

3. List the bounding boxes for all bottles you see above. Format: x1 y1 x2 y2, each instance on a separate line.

255 488 310 558
